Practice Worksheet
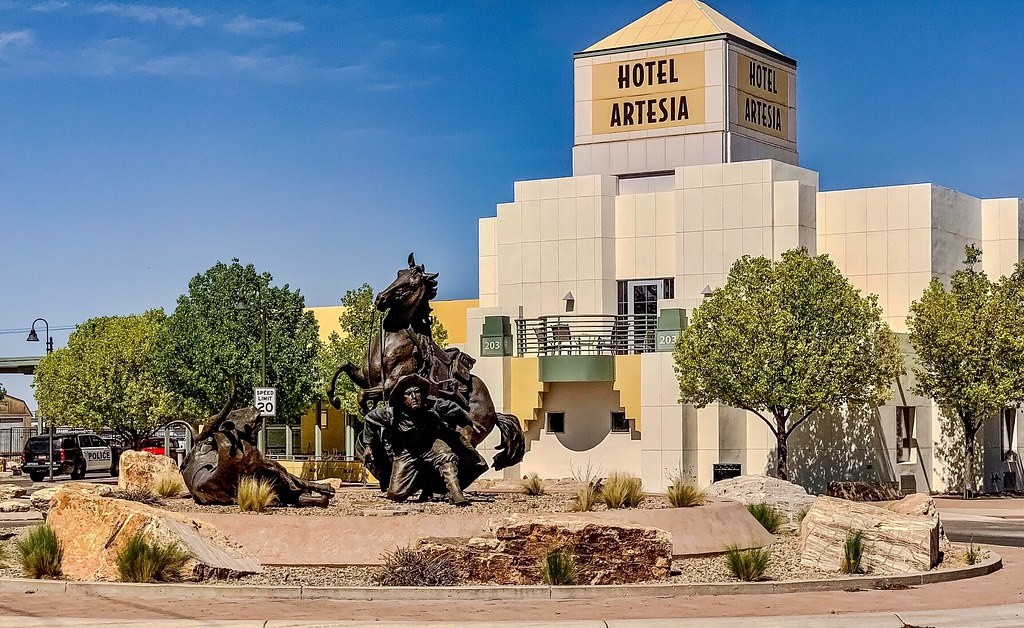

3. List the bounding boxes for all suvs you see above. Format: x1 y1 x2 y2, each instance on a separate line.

20 432 119 482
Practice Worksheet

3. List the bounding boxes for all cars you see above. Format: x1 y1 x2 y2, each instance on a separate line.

118 438 180 467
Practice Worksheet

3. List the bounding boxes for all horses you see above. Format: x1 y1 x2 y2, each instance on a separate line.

325 250 526 500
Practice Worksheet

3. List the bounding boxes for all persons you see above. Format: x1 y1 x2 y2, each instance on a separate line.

360 374 487 507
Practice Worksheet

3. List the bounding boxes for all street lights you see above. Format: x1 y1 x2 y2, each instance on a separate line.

26 318 54 483
234 281 276 460
